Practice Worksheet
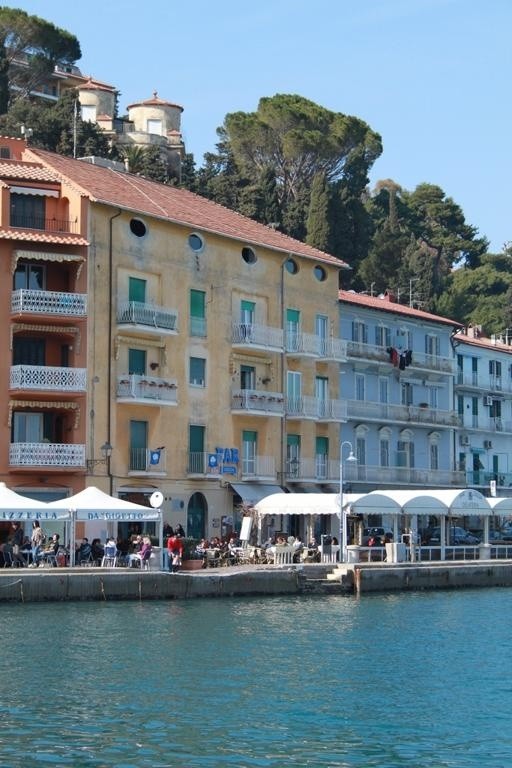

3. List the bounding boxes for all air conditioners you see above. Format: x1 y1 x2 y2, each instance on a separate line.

485 440 494 449
483 396 493 406
460 435 471 446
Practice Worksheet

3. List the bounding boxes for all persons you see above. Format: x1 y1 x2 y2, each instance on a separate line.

262 529 437 563
75 525 152 563
163 520 185 572
0 521 60 568
195 535 237 565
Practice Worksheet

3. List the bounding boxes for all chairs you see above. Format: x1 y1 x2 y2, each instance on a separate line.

1 539 151 569
200 542 321 569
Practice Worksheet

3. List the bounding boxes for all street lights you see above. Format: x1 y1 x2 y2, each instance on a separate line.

338 442 357 564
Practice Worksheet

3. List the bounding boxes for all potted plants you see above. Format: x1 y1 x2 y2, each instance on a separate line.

182 537 205 569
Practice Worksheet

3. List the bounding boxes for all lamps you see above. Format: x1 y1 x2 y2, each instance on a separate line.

87 441 113 472
277 457 302 480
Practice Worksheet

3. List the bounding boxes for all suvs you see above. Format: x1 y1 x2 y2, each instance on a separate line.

429 525 512 546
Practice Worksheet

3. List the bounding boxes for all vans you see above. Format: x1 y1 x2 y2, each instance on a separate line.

361 527 401 546
